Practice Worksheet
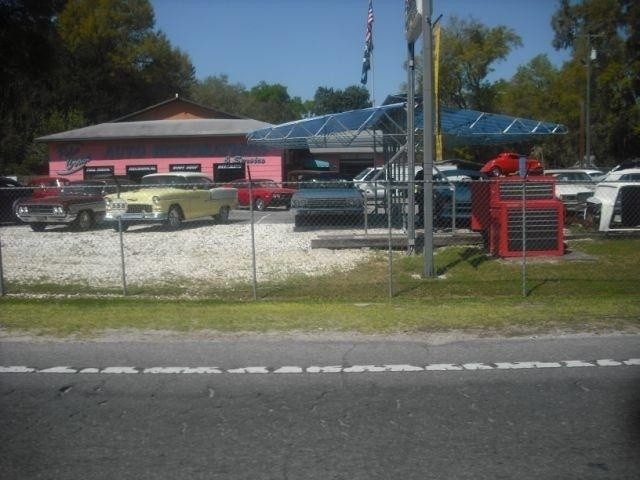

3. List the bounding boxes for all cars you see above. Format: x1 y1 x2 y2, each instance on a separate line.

217 176 294 211
10 178 117 234
434 168 488 225
0 175 42 226
102 169 240 231
290 174 365 225
23 176 71 197
542 161 640 222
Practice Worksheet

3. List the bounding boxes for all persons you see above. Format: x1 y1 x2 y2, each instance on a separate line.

415 163 424 224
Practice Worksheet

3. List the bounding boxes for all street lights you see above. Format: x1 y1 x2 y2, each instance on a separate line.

585 45 603 167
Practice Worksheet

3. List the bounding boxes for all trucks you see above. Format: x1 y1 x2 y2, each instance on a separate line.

352 163 458 203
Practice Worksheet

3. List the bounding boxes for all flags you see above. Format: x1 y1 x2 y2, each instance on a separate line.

359 0 373 83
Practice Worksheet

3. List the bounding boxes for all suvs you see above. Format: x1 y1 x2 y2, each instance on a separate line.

286 169 341 188
